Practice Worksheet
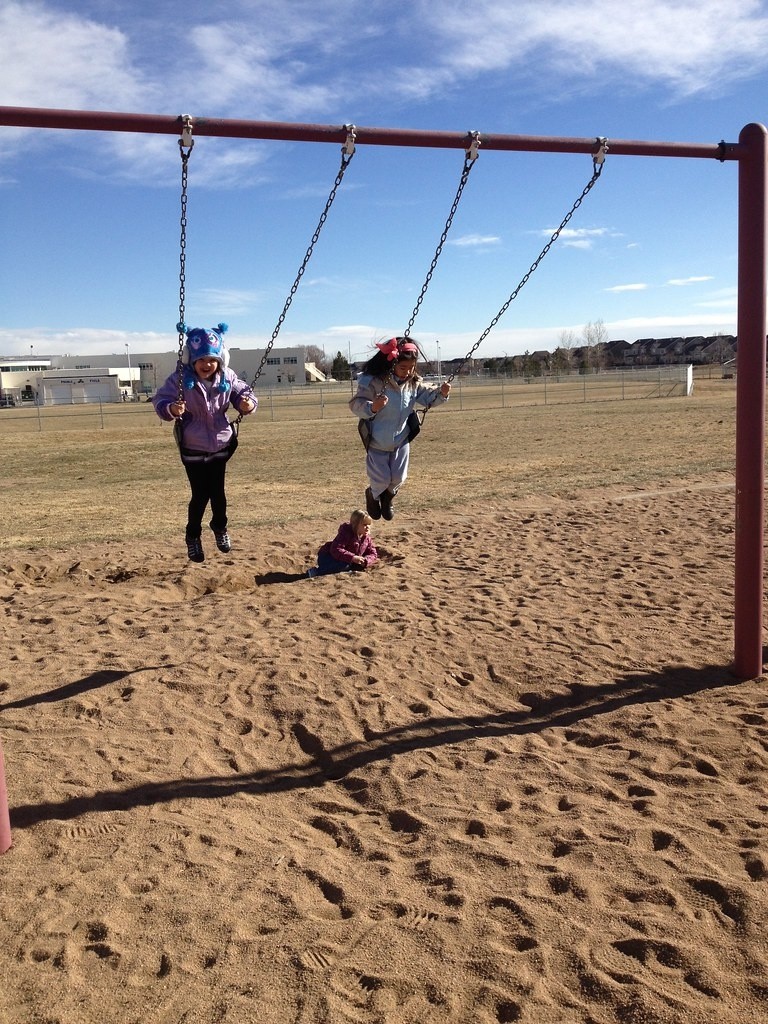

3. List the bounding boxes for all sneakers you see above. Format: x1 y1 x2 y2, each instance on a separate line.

210 520 231 553
185 535 204 562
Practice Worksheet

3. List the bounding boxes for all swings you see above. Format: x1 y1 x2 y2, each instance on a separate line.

174 136 357 468
353 149 606 457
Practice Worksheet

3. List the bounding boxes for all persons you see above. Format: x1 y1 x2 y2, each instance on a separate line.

122 389 129 401
153 322 258 563
349 337 451 520
146 390 149 397
307 510 377 577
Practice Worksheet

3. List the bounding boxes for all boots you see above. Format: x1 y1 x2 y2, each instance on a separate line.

365 486 381 520
379 488 398 521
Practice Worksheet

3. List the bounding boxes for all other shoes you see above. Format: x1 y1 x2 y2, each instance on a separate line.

307 566 318 578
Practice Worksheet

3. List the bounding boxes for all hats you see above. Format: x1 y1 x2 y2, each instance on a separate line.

176 322 230 392
